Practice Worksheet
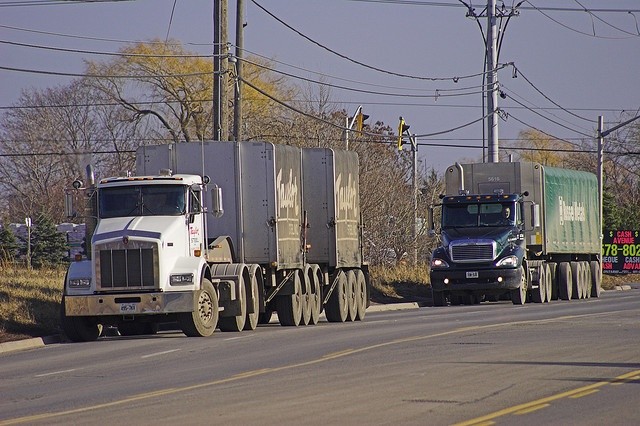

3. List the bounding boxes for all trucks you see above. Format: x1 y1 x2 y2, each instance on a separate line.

56 136 374 340
427 160 605 305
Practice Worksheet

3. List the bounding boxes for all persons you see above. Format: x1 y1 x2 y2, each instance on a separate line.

496 206 514 225
165 191 184 213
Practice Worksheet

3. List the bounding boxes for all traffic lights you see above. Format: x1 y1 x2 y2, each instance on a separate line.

357 112 370 136
396 117 411 150
